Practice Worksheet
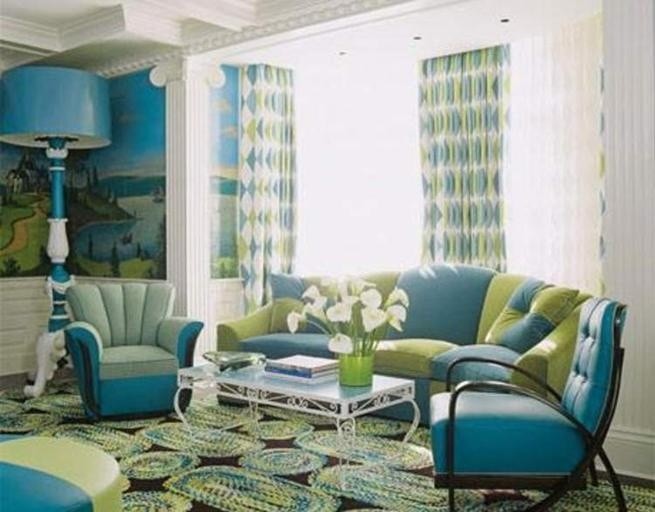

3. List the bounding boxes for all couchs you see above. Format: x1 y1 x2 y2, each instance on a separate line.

216 264 595 430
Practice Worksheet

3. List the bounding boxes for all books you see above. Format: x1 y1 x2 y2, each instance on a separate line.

264 372 338 386
265 366 340 379
266 353 340 372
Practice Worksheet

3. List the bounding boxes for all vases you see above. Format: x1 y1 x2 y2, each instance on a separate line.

337 350 375 389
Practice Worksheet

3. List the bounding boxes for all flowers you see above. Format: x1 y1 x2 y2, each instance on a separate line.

283 273 411 356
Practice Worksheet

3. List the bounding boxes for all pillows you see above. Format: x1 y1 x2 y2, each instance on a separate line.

482 276 581 354
267 271 335 335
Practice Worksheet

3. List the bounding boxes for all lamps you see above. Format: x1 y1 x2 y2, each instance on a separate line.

0 67 113 400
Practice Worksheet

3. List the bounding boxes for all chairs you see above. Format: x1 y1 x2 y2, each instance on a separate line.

426 294 630 511
62 278 205 419
